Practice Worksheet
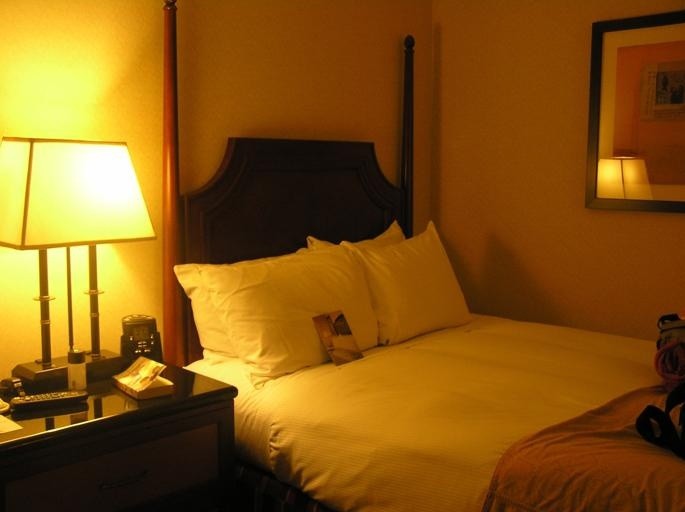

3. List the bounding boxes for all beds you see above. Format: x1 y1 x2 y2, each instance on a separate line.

163 2 685 511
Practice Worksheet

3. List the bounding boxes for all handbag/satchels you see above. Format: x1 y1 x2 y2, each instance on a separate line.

636 385 684 460
654 313 685 385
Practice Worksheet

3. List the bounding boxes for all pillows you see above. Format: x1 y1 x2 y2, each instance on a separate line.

308 219 408 248
174 247 335 365
357 220 470 348
198 246 379 388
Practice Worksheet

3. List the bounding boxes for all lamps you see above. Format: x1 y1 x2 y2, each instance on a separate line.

597 155 653 201
3 137 156 390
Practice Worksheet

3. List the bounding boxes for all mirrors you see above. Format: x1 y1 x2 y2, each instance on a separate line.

588 12 683 212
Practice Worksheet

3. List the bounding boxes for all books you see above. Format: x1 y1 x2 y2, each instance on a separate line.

111 355 176 401
311 310 365 367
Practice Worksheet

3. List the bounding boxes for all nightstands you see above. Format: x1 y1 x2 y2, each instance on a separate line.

0 364 238 511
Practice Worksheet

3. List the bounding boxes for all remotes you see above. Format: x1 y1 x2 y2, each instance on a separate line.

7 388 90 412
